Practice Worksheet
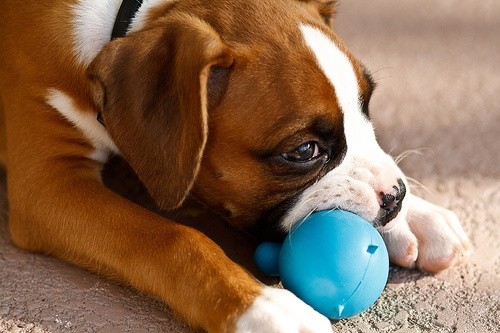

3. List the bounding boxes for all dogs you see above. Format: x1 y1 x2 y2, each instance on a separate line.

0 0 470 333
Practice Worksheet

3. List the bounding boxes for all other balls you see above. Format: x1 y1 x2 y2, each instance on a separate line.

278 209 390 321
